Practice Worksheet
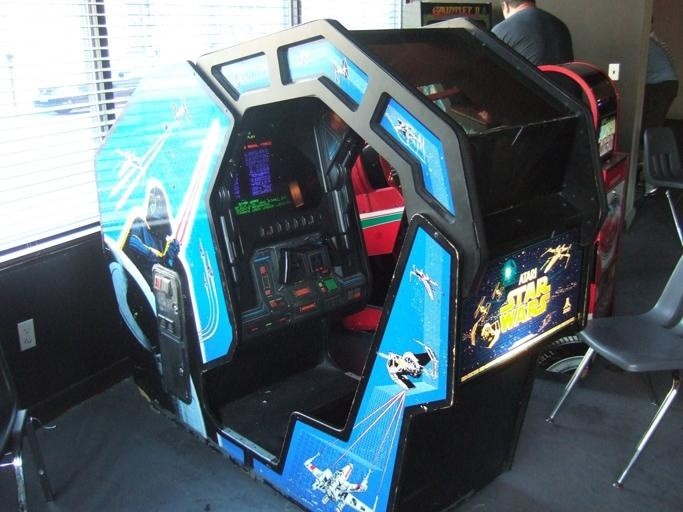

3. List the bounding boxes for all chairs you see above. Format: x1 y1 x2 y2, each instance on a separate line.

545 248 682 486
0 344 54 511
639 121 682 246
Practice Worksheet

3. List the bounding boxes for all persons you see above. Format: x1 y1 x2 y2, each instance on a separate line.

474 1 575 109
636 34 680 199
